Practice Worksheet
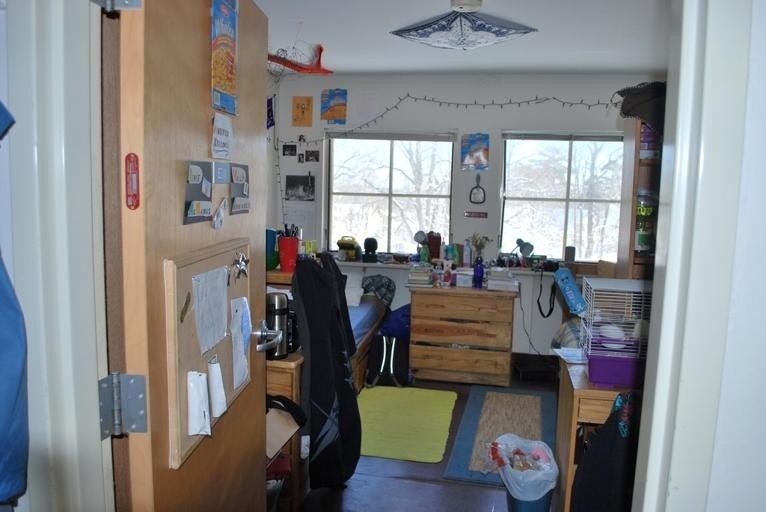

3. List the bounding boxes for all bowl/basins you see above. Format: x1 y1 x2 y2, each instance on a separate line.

267 251 279 270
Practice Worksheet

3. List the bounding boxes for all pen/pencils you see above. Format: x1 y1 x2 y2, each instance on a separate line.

280 224 298 237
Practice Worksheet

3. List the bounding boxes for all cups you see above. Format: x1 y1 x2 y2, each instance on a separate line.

278 236 298 272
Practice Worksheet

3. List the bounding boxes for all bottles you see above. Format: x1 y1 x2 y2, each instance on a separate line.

636 192 654 216
439 239 471 268
474 257 484 288
267 293 288 358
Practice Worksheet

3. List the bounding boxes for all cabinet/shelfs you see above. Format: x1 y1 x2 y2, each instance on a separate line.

406 282 519 388
617 117 665 279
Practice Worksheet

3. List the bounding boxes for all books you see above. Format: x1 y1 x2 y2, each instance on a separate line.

488 266 520 292
404 263 435 287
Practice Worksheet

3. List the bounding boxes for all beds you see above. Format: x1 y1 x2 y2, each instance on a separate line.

266 294 385 469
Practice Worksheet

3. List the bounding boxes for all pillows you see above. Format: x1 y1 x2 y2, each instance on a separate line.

339 271 365 307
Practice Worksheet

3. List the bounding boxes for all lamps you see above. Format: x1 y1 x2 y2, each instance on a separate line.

506 237 534 272
387 9 539 55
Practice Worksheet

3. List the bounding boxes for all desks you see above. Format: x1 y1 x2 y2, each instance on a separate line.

554 352 634 511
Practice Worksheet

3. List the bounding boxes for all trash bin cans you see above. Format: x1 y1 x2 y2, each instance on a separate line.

497 440 559 512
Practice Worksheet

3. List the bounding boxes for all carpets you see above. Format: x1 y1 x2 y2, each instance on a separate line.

357 384 558 492
